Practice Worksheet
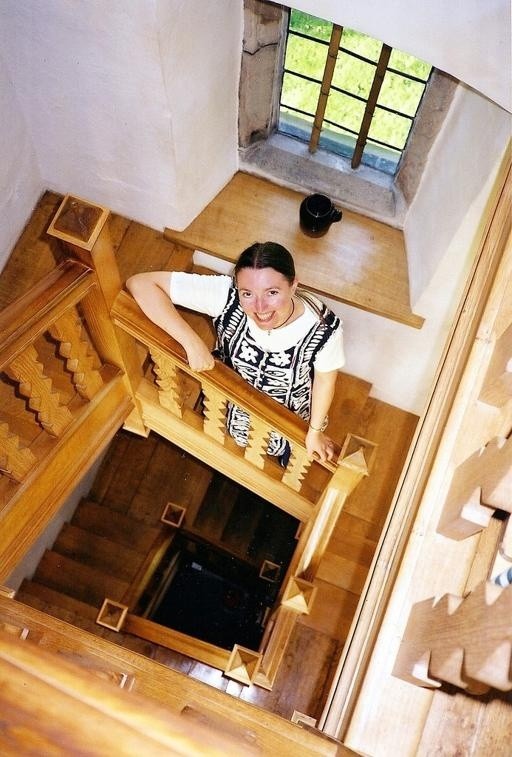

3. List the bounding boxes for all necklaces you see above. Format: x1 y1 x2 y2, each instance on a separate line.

268 298 294 336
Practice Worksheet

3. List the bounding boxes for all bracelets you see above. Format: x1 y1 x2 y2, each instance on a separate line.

309 424 328 433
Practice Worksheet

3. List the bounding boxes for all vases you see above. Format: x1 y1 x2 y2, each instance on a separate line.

302 191 342 241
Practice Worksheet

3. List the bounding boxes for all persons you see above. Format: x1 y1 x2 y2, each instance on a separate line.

126 241 346 469
